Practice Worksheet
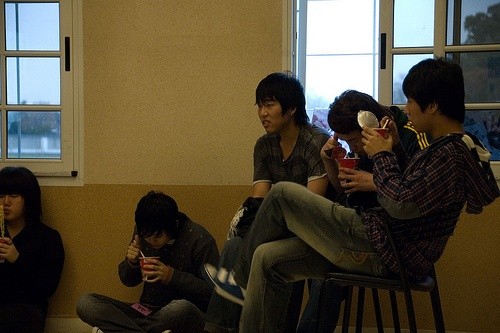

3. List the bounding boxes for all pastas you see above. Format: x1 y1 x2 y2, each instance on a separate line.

0 205 6 239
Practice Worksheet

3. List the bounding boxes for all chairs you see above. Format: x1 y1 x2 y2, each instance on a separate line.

317 195 466 333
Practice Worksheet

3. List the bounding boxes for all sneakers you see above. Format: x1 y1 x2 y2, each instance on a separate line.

204 263 244 306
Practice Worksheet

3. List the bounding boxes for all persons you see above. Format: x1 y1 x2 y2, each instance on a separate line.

199 57 485 333
76 189 220 333
276 90 434 333
201 71 349 332
0 165 67 333
